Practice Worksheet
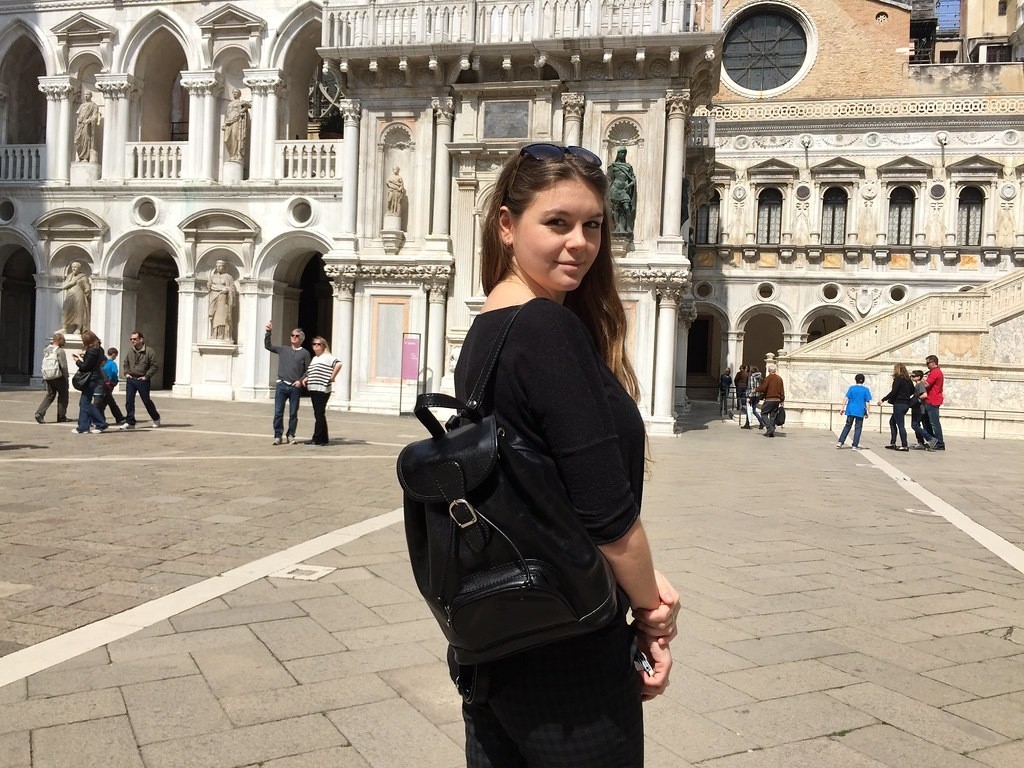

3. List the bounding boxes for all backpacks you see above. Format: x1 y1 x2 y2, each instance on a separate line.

41 346 63 383
396 299 619 694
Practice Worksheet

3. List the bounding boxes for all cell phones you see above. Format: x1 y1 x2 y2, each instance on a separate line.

635 649 654 678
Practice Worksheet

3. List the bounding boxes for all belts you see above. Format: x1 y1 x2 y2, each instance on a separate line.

276 379 292 385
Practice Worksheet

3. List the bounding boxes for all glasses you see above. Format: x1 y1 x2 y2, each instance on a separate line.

507 144 602 206
910 375 918 378
130 338 141 341
926 361 934 366
291 333 301 338
312 343 322 346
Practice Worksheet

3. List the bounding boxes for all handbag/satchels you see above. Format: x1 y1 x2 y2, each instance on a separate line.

775 404 786 428
910 395 921 408
72 369 93 391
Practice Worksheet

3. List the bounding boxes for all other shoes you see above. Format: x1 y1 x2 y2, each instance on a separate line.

287 435 297 444
153 417 161 428
35 414 45 424
92 426 108 433
119 423 135 430
57 417 73 422
837 438 944 452
116 416 126 423
72 428 90 434
720 410 774 438
304 440 316 444
273 438 282 445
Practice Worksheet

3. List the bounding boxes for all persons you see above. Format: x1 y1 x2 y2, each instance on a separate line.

688 227 697 271
734 363 785 437
34 333 73 424
264 321 312 445
719 368 732 411
837 374 872 450
54 262 91 334
71 329 126 434
877 355 946 452
445 142 682 768
607 145 636 234
74 90 99 162
222 86 252 161
303 336 343 446
119 332 161 428
206 259 236 339
386 165 404 217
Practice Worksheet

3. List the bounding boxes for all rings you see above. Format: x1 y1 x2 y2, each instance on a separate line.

671 614 674 623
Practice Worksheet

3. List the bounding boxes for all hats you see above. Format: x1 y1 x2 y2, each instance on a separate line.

855 374 865 382
